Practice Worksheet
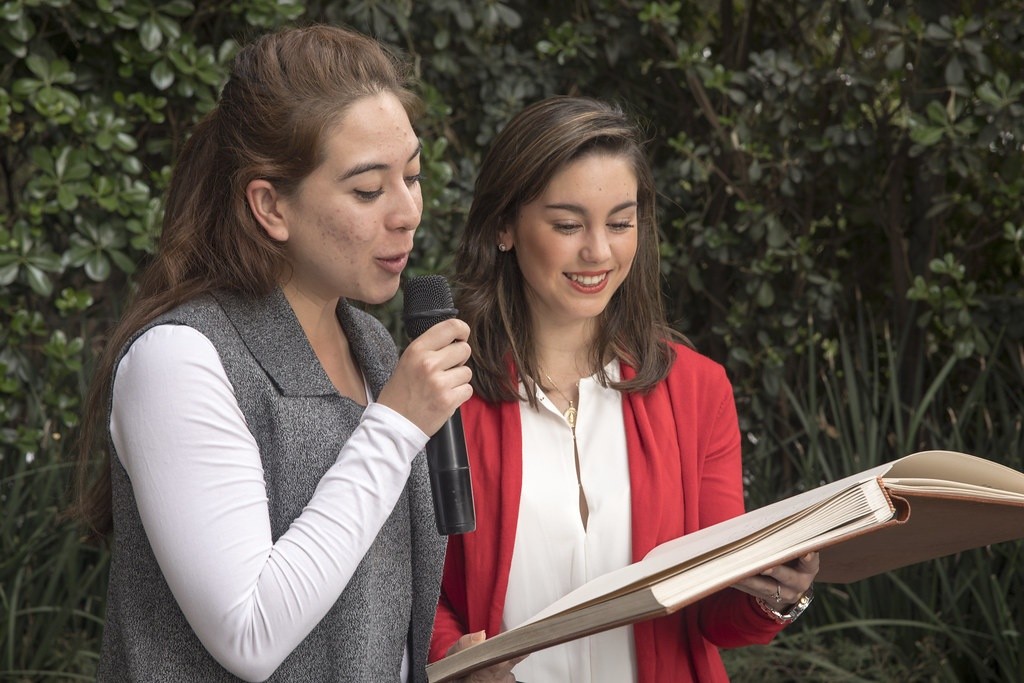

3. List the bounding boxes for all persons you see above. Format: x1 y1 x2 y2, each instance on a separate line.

426 96 823 683
73 25 473 683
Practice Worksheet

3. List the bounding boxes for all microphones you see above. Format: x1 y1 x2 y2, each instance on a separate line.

400 275 478 534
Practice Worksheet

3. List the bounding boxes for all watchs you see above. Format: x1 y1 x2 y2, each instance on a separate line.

755 582 816 625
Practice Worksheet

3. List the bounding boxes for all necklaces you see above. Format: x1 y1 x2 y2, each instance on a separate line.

539 366 583 427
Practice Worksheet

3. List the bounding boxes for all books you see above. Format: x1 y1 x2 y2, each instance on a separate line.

426 450 1023 683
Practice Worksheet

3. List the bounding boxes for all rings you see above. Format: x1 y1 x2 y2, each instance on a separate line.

771 585 783 603
